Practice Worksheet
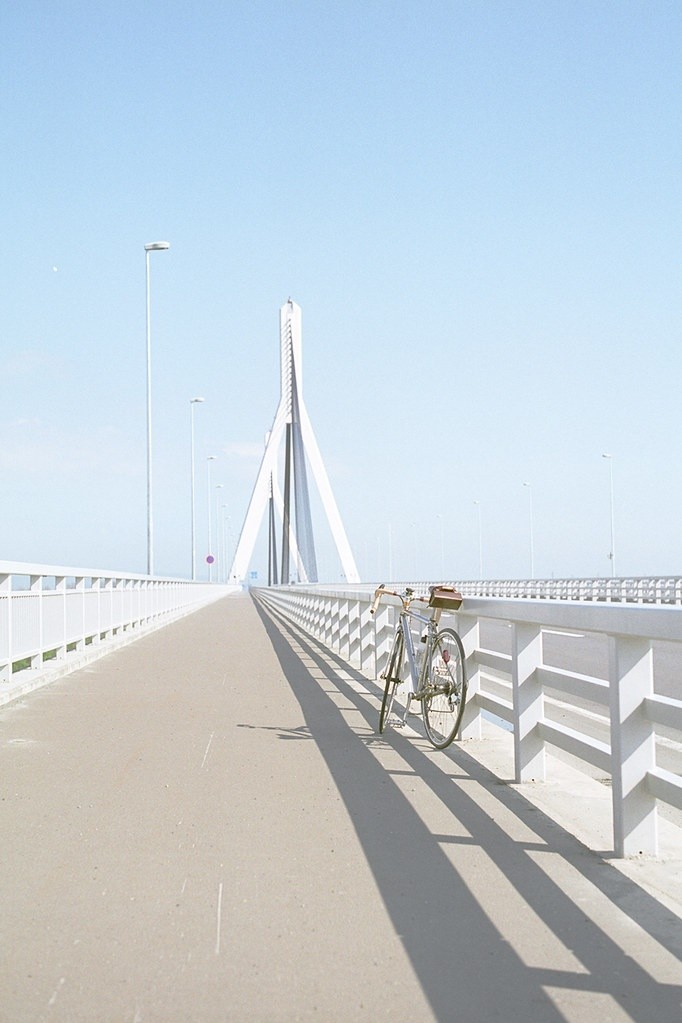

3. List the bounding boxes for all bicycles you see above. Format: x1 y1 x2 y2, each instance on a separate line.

368 583 469 750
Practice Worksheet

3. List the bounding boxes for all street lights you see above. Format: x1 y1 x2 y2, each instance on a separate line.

601 450 616 580
471 500 482 581
522 481 534 581
221 503 233 582
215 484 224 583
189 396 206 581
206 454 218 583
436 513 446 581
144 239 170 580
